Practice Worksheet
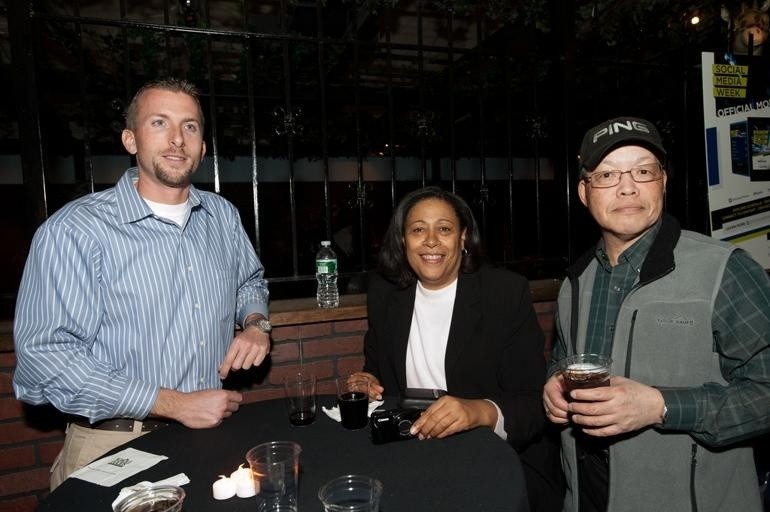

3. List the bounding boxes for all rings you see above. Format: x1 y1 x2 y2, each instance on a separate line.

545 410 552 416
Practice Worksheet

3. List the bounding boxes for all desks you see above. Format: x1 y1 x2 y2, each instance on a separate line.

30 392 530 512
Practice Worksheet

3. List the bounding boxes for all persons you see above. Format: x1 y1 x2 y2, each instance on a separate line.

11 79 271 492
344 188 565 512
541 116 770 512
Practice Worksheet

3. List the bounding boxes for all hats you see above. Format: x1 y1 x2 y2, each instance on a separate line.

580 114 668 171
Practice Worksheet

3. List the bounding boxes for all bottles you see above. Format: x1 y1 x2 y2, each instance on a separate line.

315 240 340 308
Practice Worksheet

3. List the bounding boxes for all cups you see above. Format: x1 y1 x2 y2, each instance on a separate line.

245 440 303 512
558 354 615 431
282 372 318 426
115 485 186 512
316 474 384 511
334 374 369 429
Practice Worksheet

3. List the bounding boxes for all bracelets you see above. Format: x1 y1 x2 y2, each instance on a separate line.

662 407 670 423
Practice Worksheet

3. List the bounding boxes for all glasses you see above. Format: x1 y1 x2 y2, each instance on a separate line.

583 164 665 188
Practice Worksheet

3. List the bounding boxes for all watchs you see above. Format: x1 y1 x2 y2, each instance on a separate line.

246 317 272 334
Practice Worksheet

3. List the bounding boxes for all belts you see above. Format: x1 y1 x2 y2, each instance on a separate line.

72 413 173 432
